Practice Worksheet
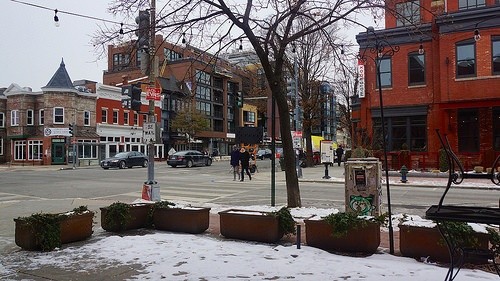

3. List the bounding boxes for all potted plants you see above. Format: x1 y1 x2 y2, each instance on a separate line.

144 200 211 233
219 205 298 243
398 212 500 264
304 209 397 253
13 205 94 255
99 201 151 235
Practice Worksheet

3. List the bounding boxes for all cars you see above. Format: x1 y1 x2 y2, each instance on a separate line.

304 151 322 165
251 149 272 160
167 150 212 168
100 151 149 170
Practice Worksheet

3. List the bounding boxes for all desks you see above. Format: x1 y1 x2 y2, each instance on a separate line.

424 128 500 281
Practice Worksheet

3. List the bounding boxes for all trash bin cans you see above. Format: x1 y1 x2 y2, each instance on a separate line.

343 156 383 217
439 149 451 172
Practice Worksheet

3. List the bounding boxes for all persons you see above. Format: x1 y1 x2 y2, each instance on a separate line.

230 145 240 181
336 145 344 166
239 147 252 182
202 149 208 155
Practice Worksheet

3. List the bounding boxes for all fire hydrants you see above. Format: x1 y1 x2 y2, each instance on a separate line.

399 165 408 183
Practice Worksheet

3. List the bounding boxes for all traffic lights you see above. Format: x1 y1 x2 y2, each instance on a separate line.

68 123 75 136
131 84 142 111
235 90 243 108
134 9 150 51
